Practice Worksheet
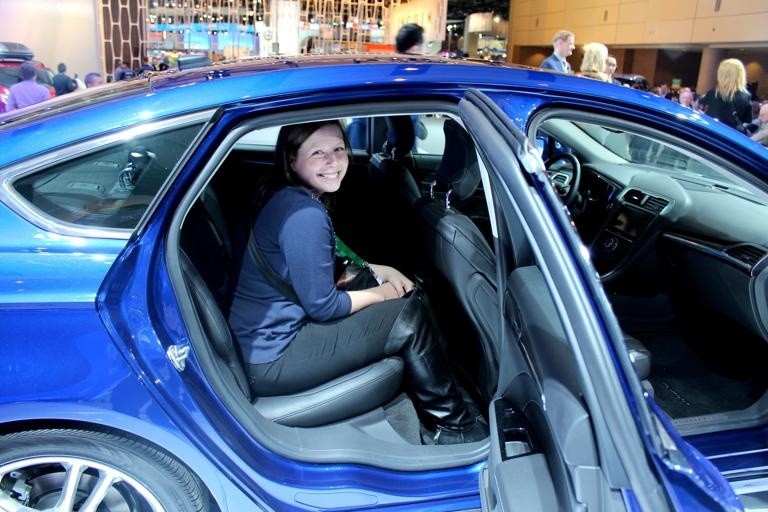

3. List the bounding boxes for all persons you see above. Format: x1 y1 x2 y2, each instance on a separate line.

538 28 767 152
346 23 430 152
228 119 486 444
0 52 175 114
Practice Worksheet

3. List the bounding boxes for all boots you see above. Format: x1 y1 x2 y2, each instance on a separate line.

378 285 490 446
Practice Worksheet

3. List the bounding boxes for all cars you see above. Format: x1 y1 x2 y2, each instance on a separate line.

0 42 55 115
0 54 768 511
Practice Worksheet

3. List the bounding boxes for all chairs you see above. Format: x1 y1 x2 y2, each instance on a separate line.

419 119 655 409
122 151 406 423
368 113 427 207
